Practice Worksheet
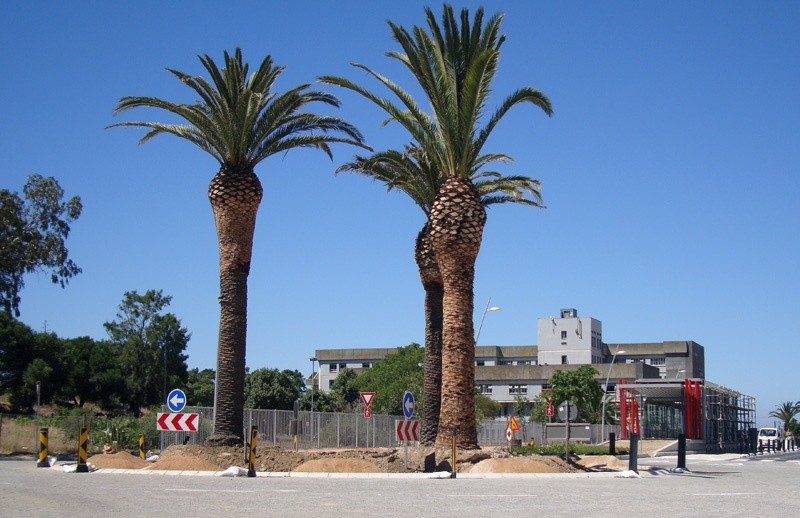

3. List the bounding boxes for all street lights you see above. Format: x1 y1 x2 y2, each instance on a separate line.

309 357 318 442
474 296 502 345
675 368 686 380
601 343 625 443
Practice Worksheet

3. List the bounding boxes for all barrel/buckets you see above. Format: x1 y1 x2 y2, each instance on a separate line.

511 439 521 449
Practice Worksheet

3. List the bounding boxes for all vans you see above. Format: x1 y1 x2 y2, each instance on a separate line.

757 427 780 451
